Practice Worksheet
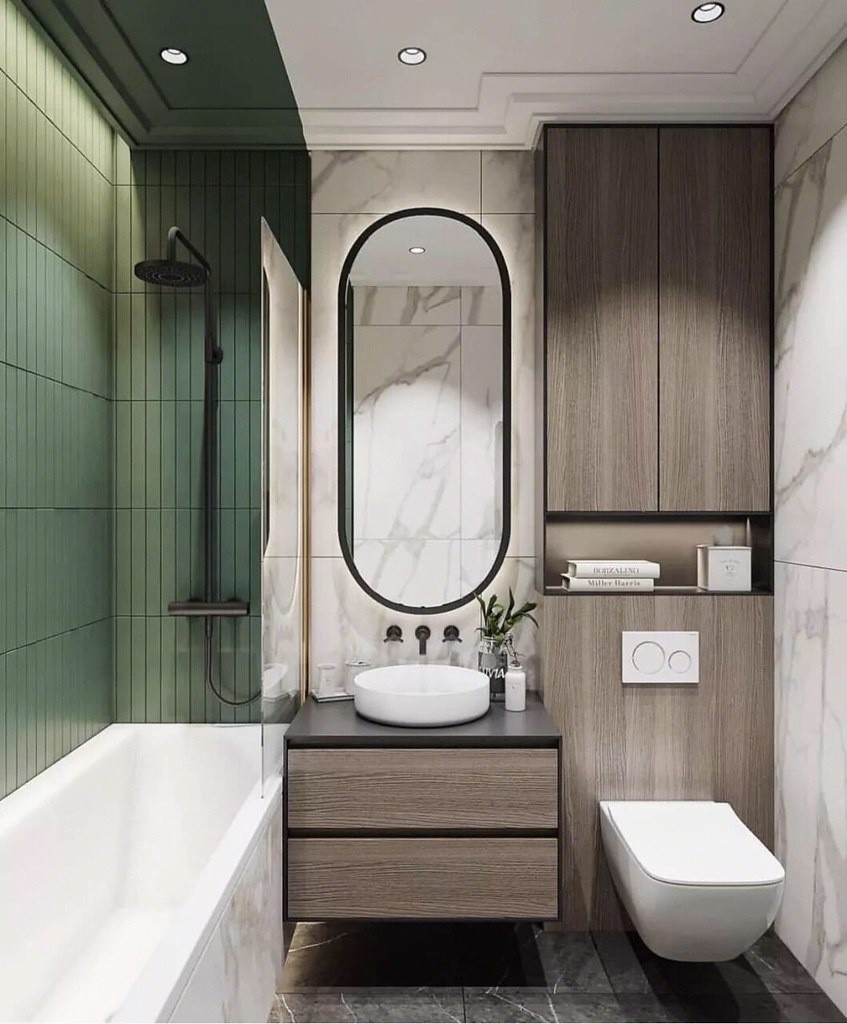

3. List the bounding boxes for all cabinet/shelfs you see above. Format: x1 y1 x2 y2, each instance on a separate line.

528 124 776 930
280 699 566 927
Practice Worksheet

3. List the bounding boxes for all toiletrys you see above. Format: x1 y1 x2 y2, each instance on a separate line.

503 652 531 712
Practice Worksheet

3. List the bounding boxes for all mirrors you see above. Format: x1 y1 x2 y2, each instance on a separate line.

334 207 514 618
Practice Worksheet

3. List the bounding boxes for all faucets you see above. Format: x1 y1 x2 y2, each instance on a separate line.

415 626 431 657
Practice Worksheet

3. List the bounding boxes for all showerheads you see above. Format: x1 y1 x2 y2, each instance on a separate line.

131 258 210 291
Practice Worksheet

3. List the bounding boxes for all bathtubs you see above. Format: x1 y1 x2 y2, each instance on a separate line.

0 717 283 1024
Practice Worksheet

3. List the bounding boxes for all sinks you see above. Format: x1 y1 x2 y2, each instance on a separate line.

352 662 493 729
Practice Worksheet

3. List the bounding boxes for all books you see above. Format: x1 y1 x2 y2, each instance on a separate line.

560 574 655 593
566 558 661 579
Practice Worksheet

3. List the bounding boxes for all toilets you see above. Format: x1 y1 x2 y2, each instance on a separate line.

600 795 788 968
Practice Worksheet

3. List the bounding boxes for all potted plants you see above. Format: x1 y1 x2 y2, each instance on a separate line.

470 585 541 702
